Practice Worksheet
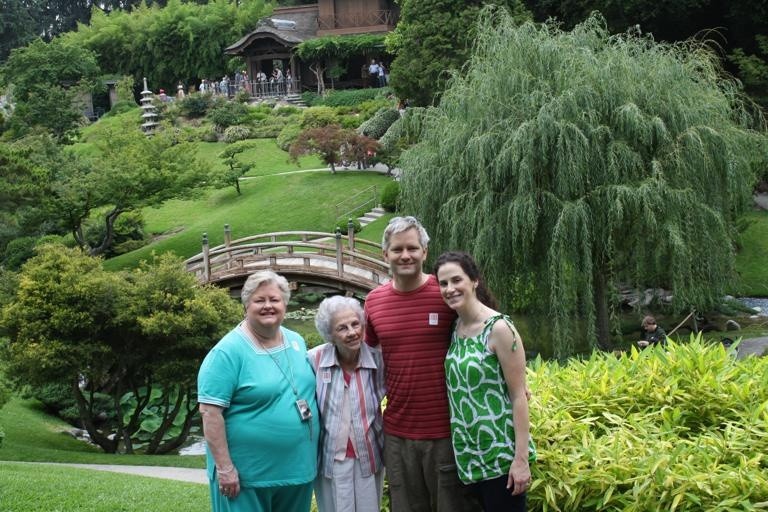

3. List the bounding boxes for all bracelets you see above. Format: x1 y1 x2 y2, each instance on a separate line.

213 464 236 474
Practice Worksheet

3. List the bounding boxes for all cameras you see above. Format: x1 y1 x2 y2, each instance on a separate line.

296 400 313 422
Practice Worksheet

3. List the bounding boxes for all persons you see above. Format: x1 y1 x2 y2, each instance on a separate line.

368 58 378 88
196 264 321 512
378 61 386 87
306 294 386 512
432 249 532 512
636 316 666 349
157 67 291 101
363 216 532 512
